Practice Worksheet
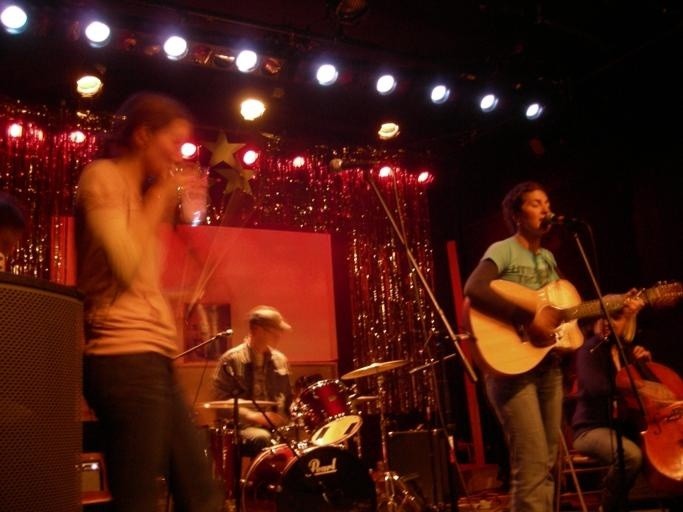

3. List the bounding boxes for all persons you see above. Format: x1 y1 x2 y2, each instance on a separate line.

72 92 226 511
562 299 651 512
214 306 294 433
461 182 645 512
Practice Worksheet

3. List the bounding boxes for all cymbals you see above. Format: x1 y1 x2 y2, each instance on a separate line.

204 398 277 409
342 359 411 380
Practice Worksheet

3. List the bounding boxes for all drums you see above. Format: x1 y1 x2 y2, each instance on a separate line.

240 444 378 512
290 378 363 444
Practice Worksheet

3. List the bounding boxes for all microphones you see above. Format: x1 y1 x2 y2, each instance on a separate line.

329 159 379 171
217 329 233 337
544 212 586 225
222 362 245 391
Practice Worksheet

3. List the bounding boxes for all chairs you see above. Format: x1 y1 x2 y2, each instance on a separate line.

557 416 617 512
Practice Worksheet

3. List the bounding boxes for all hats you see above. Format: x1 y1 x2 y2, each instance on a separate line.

248 304 292 332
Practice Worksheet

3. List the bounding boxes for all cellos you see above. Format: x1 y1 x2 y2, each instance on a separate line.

610 332 683 483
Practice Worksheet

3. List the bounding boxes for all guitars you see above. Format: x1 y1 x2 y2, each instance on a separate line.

461 279 683 379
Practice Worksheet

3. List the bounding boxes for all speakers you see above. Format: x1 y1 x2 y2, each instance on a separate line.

0 270 84 512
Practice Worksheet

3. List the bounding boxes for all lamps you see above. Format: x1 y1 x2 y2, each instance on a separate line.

317 64 338 87
379 121 399 140
373 72 396 96
241 98 267 121
2 5 27 34
525 102 544 120
477 94 499 112
234 40 258 74
87 20 110 48
431 82 452 105
164 33 187 60
